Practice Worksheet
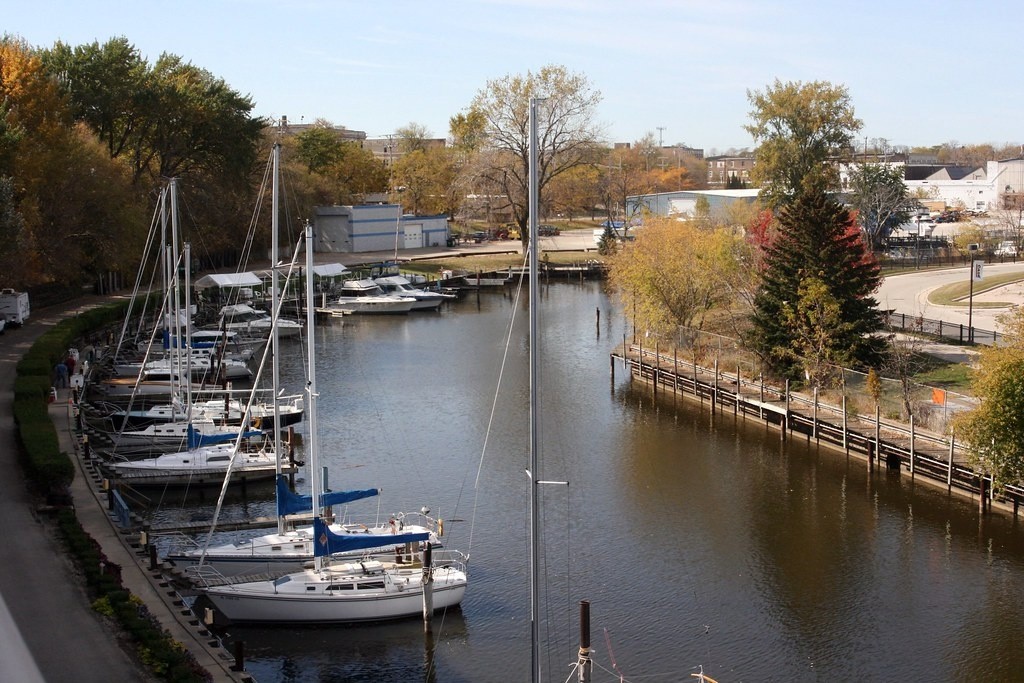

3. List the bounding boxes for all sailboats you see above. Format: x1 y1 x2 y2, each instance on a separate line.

72 137 723 683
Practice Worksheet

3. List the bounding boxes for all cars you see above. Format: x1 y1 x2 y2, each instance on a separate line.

470 222 562 243
931 206 988 223
